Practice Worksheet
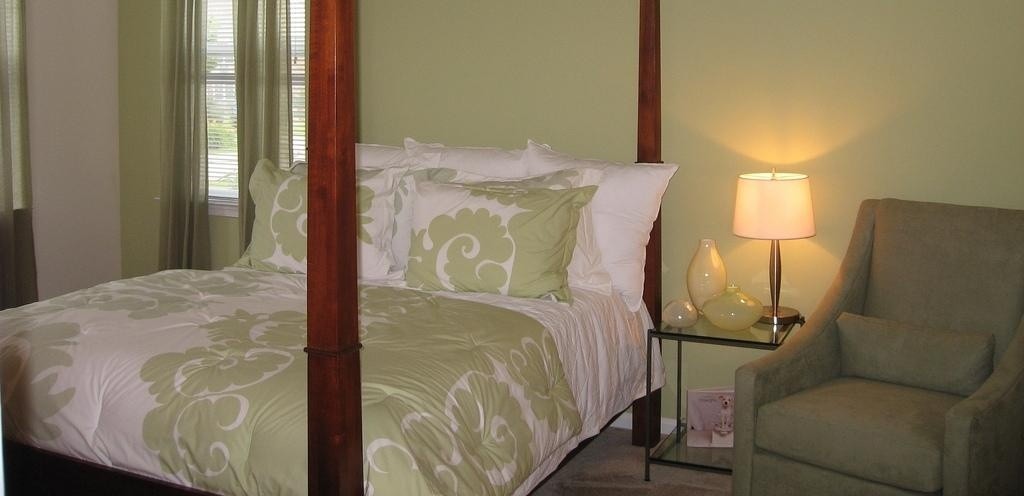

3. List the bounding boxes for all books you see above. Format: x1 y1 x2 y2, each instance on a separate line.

684 387 736 451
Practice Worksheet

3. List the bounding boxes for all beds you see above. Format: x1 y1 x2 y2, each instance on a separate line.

0 160 658 495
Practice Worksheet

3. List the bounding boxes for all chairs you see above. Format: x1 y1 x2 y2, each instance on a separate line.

732 197 1024 496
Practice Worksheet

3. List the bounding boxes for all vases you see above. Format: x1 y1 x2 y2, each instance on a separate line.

686 239 763 332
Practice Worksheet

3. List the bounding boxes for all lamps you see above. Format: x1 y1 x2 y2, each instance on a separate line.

731 172 817 327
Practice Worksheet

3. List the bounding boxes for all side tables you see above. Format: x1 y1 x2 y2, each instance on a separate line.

643 311 796 482
